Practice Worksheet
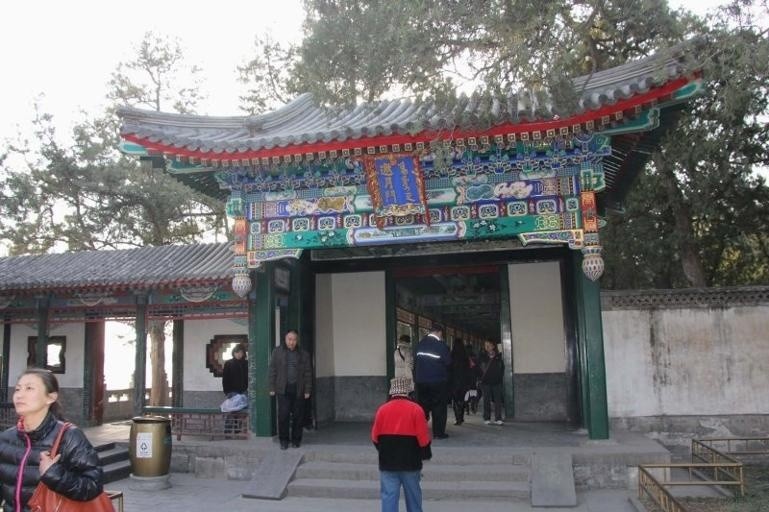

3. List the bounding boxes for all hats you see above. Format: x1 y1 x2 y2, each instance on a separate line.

388 375 412 397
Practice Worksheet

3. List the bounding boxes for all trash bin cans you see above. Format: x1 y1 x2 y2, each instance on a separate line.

130 418 172 477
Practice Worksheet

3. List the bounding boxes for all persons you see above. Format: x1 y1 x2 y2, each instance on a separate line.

267 329 313 451
392 323 505 440
222 343 249 440
0 367 105 512
370 376 433 511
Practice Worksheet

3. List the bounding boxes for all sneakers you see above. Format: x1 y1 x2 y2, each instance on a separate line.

454 412 477 425
484 419 504 426
280 443 300 450
432 432 450 439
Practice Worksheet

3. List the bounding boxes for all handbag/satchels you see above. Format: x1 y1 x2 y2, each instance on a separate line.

26 421 116 512
